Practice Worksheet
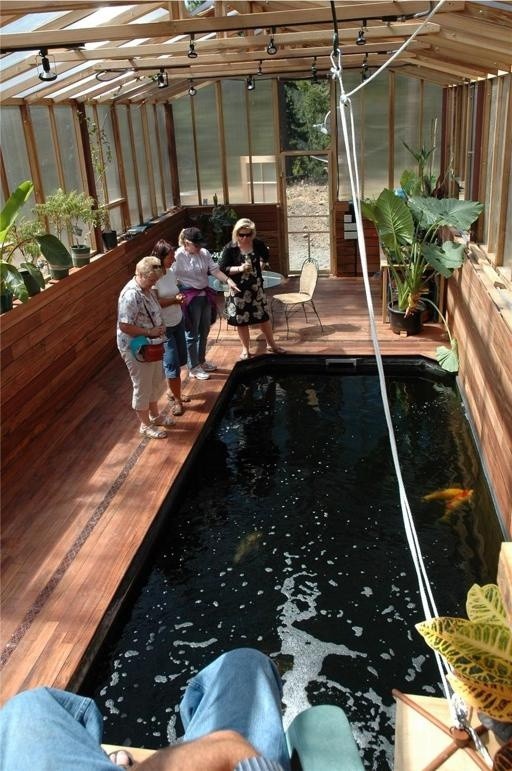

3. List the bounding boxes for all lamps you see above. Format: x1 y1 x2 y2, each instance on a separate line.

267 36 277 55
357 32 367 45
247 75 254 90
361 63 374 84
257 59 263 75
188 39 197 58
37 47 58 81
189 79 196 97
157 67 168 89
311 56 322 87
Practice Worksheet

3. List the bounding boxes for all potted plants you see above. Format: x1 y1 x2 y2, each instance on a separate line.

30 188 84 280
0 179 73 315
0 215 55 296
64 191 110 268
351 187 484 373
73 82 126 250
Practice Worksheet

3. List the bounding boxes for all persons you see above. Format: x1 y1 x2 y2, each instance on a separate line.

217 218 288 361
0 647 293 771
116 256 177 439
151 240 191 416
170 227 242 381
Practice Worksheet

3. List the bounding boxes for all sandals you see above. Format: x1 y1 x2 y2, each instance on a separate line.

171 401 183 415
148 412 176 426
138 423 167 439
166 391 190 402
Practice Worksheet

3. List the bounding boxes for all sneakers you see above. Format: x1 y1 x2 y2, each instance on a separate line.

188 365 211 380
200 362 218 371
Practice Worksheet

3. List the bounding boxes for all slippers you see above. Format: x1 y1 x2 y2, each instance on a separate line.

265 347 288 355
240 352 251 360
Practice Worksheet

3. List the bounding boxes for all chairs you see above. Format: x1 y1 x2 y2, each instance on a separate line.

270 257 325 341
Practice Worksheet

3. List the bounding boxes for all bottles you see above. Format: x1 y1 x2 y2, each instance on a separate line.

212 192 218 205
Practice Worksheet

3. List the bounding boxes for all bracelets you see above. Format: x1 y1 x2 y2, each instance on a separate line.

237 266 239 274
144 328 149 337
224 277 230 284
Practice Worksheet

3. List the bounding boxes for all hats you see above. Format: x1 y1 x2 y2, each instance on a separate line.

183 227 207 246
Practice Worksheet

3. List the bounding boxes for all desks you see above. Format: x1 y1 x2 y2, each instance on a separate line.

207 270 284 343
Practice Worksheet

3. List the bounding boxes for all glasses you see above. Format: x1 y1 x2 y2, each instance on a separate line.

238 232 253 237
152 265 162 269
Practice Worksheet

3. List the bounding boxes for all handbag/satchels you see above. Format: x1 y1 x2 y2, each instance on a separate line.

161 303 183 328
142 344 165 363
